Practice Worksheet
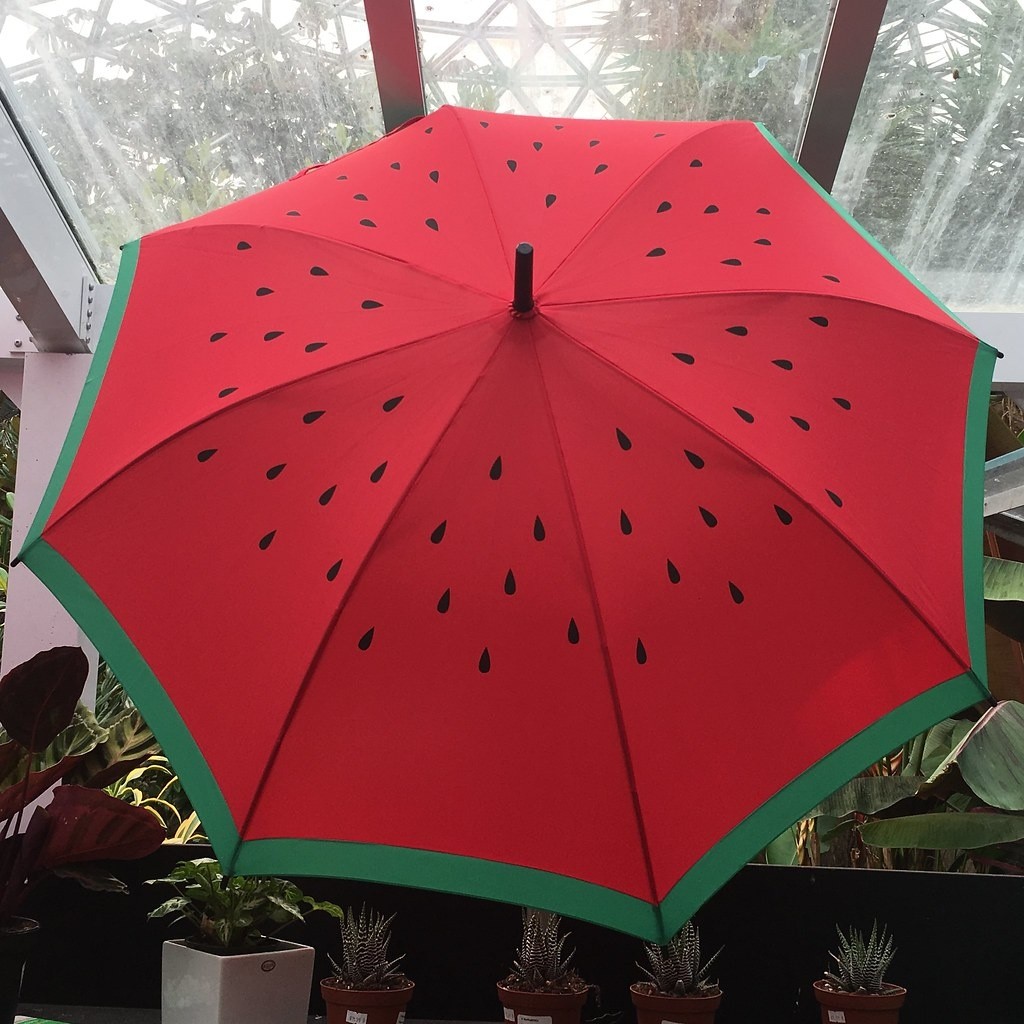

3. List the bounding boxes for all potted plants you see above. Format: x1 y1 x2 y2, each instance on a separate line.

812 918 906 1024
497 907 589 1024
320 901 415 1024
630 920 725 1024
139 858 343 1024
0 640 165 1024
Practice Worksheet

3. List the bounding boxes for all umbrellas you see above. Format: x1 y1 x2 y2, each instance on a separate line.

12 104 1003 960
